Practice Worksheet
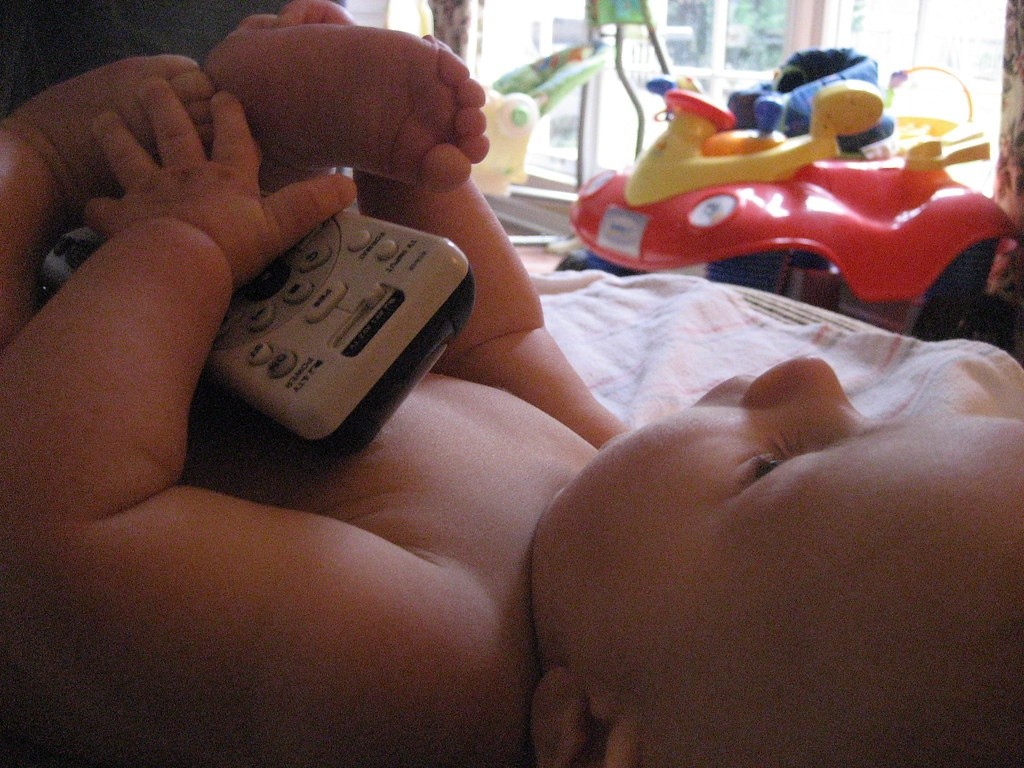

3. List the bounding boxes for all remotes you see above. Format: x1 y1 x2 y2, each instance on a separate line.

39 189 478 457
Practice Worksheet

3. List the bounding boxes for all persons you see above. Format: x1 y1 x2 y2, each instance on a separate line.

0 1 1024 768
